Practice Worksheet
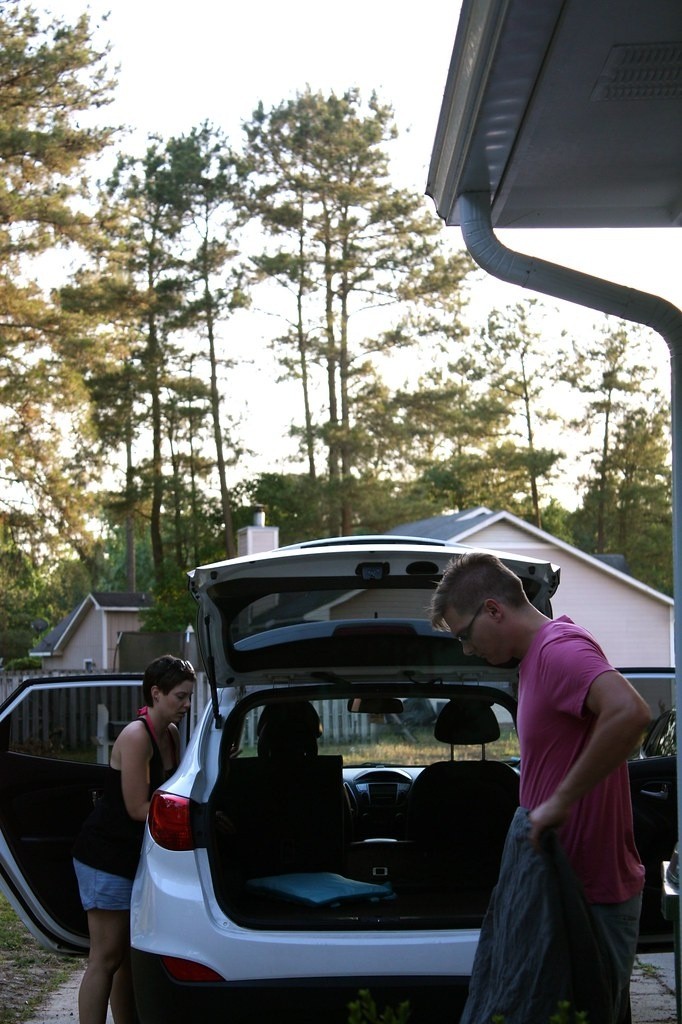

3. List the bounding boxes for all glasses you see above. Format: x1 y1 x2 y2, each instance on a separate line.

177 660 195 673
456 604 484 642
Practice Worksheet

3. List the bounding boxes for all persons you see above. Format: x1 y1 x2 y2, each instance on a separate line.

430 553 652 1024
70 656 196 1024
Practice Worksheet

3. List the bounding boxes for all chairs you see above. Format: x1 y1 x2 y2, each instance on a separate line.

396 696 519 885
232 701 351 867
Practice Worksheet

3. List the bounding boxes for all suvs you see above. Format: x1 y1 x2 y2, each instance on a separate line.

0 535 677 1024
